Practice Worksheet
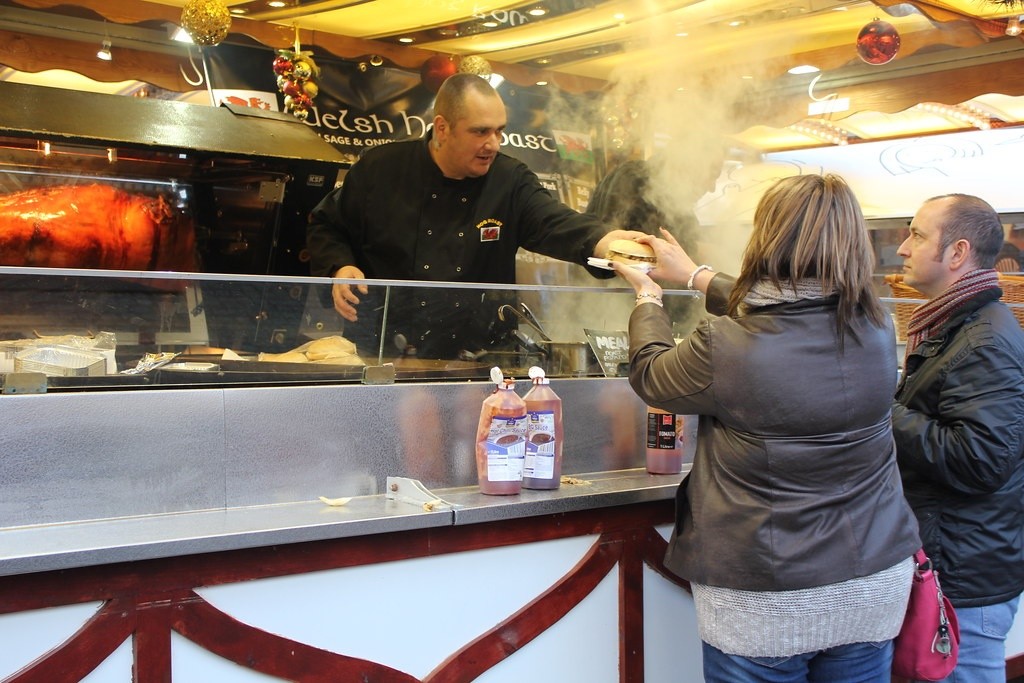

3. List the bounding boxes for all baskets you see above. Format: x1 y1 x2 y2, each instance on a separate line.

882 274 1024 341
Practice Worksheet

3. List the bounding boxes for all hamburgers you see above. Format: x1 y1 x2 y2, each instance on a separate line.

607 239 657 268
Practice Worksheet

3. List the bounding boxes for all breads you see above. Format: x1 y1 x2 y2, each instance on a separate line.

260 352 308 363
305 337 355 360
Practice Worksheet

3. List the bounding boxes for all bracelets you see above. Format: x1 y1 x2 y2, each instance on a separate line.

634 292 665 306
688 266 714 297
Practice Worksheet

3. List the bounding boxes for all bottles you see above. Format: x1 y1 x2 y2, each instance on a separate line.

475 367 527 495
645 405 683 474
522 366 564 489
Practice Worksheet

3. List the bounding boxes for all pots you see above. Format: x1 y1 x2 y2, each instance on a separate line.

513 330 597 372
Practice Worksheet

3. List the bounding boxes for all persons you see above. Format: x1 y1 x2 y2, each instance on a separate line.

893 198 1024 683
608 174 923 683
567 125 729 330
309 73 648 366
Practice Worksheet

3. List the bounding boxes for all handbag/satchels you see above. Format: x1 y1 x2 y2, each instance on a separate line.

891 569 960 682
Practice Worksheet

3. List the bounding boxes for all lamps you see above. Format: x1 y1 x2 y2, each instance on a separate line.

856 1 900 65
370 55 383 65
182 0 231 48
455 55 491 84
165 20 195 44
96 19 112 60
1005 15 1022 36
